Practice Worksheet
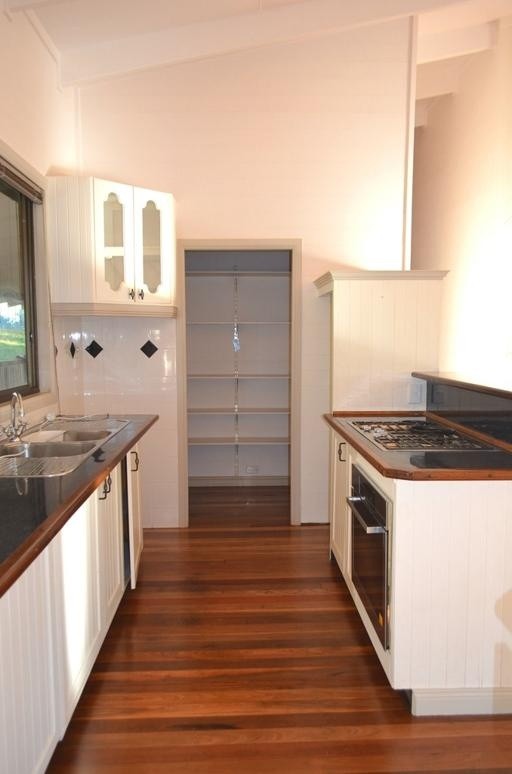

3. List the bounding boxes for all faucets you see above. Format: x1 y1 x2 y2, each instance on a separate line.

9 455 28 496
10 390 25 427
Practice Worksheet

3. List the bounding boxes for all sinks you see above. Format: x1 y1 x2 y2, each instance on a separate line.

20 419 131 443
0 443 100 478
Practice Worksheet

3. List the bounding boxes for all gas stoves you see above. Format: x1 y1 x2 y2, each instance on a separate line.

346 419 493 452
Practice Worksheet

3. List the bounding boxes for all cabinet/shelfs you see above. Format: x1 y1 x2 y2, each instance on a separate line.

66 462 122 668
332 433 351 575
48 176 179 315
183 270 289 488
121 442 144 592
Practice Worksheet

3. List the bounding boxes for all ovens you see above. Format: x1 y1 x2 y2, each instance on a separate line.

345 463 392 651
